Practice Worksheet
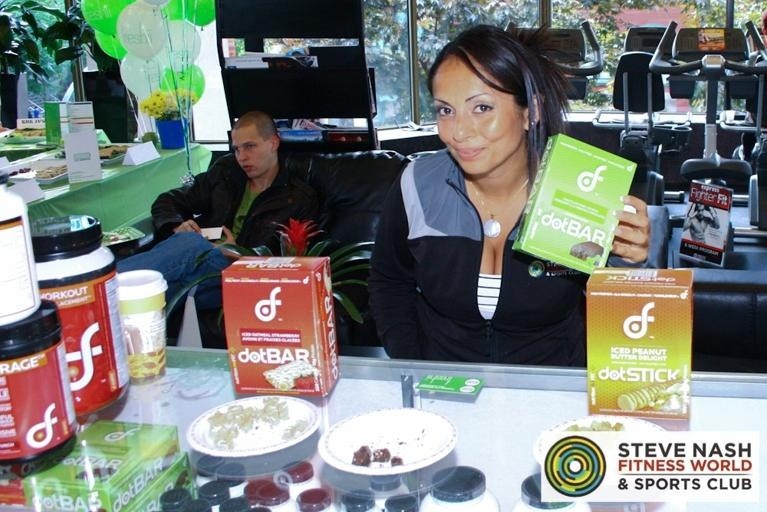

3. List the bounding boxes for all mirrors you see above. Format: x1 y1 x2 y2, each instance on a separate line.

0 0 85 168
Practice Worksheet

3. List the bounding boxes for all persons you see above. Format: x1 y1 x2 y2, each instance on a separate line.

683 203 720 244
367 24 650 370
115 111 321 313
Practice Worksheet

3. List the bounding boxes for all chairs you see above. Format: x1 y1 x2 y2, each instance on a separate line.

207 150 410 343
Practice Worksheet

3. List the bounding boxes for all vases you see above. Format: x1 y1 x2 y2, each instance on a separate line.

155 118 185 149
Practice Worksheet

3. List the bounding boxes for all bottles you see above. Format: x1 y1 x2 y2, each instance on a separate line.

158 453 592 512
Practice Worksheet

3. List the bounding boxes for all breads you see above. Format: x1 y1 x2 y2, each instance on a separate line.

98 144 129 159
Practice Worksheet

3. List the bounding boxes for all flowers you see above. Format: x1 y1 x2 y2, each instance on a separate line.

139 87 198 120
166 217 376 324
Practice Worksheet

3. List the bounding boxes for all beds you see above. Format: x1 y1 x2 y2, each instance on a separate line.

0 141 214 234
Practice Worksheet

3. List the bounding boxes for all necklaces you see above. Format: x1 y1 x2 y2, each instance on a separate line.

471 178 530 238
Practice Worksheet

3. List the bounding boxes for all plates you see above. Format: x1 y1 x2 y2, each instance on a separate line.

318 406 453 479
185 394 319 458
531 412 668 467
101 226 145 247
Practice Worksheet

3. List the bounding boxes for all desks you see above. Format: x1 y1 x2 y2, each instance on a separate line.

1 345 766 512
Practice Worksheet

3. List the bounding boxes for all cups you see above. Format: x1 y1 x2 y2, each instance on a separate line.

116 266 169 386
